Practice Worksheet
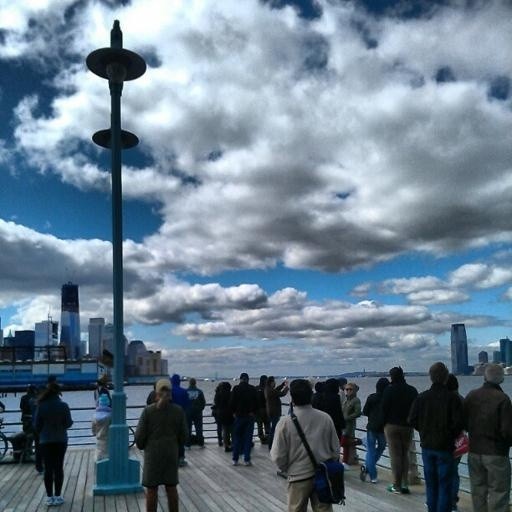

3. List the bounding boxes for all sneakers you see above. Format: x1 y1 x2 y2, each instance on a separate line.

185 446 190 450
199 444 206 448
386 484 402 495
371 478 379 484
233 459 239 466
244 461 253 465
360 463 368 481
35 463 43 472
341 461 350 471
46 497 52 506
401 487 409 494
53 496 64 505
179 460 187 467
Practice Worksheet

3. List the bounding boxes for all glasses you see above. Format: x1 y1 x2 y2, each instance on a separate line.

346 388 353 391
270 380 275 382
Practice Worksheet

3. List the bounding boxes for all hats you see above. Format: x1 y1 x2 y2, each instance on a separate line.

449 374 458 391
46 382 64 398
156 378 173 393
98 373 108 384
99 393 111 407
240 372 249 379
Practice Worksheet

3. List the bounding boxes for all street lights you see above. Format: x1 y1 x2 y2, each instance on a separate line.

86 20 147 497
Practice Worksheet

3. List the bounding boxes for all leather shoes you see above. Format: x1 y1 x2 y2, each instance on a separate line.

219 443 223 446
250 442 254 447
228 441 232 444
225 447 233 452
260 437 268 442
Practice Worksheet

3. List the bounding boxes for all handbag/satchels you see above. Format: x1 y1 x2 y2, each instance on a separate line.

453 434 470 458
225 387 244 432
289 407 345 503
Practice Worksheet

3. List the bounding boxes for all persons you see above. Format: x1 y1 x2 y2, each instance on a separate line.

182 377 207 449
264 375 290 453
461 364 512 512
384 366 418 494
31 381 73 506
170 374 190 468
229 372 262 467
311 381 327 409
311 377 342 442
337 377 346 414
405 362 463 512
212 382 232 451
91 378 113 409
338 383 360 466
358 377 387 484
270 379 340 512
19 384 40 458
255 375 272 445
135 378 190 512
91 393 112 459
146 380 160 405
448 374 466 504
